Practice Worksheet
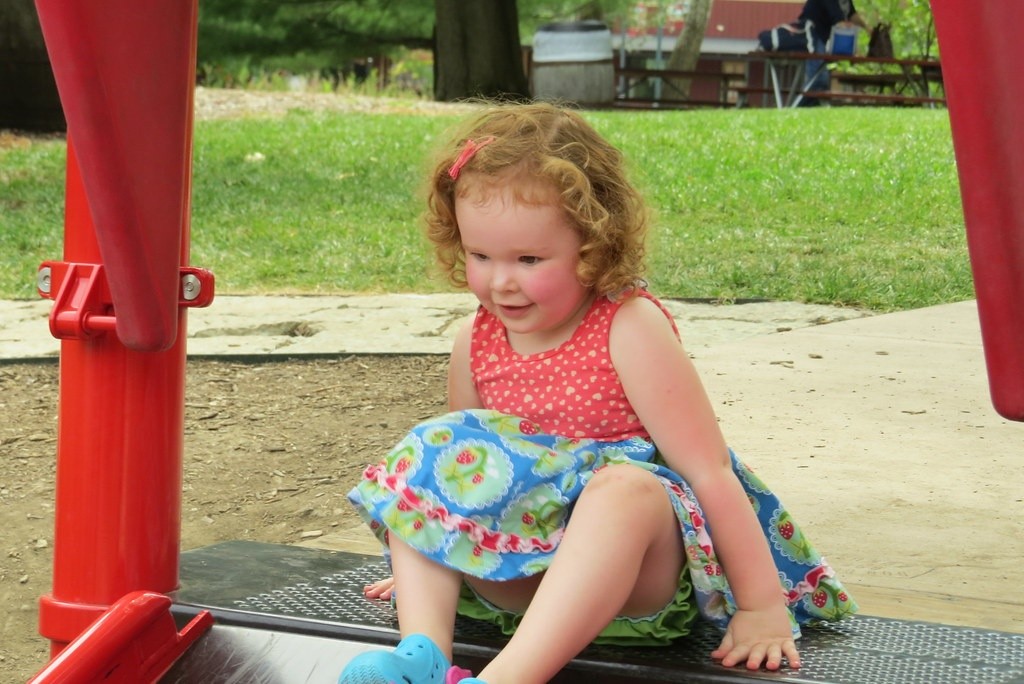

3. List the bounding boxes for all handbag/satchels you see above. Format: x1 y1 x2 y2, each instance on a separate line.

865 22 895 58
758 18 817 50
826 24 860 56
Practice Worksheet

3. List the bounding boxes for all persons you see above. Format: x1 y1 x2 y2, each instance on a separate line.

337 102 859 684
799 0 872 91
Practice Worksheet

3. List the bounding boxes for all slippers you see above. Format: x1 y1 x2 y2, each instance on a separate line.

336 633 489 683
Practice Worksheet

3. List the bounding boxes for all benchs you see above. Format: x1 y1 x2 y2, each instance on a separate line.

731 85 804 108
804 95 943 109
612 67 745 106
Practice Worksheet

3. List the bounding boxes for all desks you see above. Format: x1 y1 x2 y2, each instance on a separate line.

748 49 945 109
